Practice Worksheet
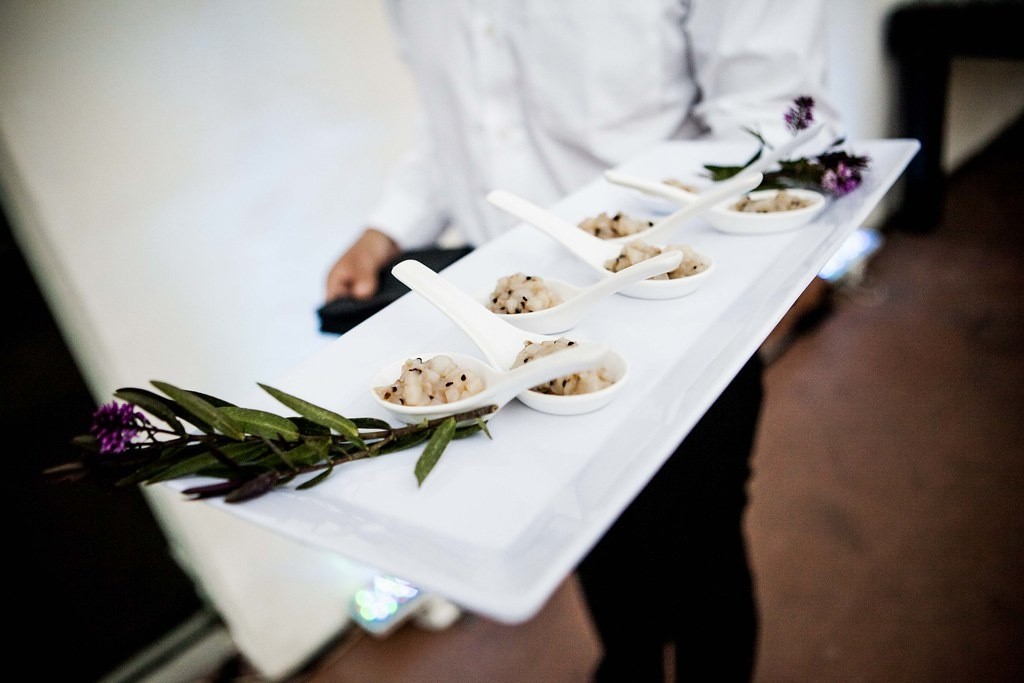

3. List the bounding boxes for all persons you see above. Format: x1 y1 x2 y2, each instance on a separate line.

327 0 865 683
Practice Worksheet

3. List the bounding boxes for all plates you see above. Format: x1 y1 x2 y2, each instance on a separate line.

168 136 919 626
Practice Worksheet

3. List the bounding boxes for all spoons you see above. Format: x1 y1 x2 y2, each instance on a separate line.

390 258 628 417
480 248 681 334
662 123 834 192
371 341 612 429
485 187 714 301
602 162 824 236
576 172 763 249
